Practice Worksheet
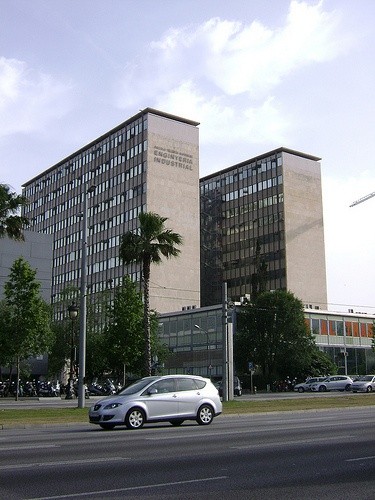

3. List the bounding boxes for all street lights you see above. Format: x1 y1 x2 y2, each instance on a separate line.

78 184 98 408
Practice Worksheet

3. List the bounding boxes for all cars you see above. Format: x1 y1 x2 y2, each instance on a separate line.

89 374 223 430
207 374 243 397
293 376 326 393
310 375 353 392
351 375 375 393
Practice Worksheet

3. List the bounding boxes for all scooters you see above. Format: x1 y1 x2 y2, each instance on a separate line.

270 375 307 393
0 377 125 400
